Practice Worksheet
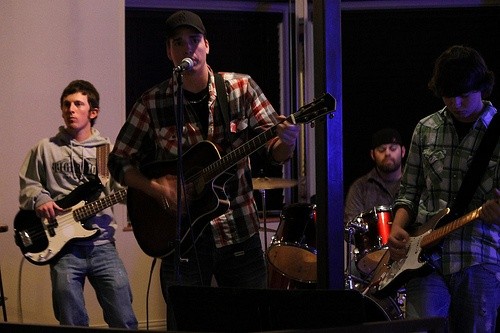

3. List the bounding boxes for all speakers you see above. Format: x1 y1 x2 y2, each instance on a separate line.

168 286 449 333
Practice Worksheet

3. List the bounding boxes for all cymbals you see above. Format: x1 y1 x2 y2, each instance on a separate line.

253 178 297 189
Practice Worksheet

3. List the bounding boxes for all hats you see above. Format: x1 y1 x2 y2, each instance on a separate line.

161 11 206 42
370 129 403 150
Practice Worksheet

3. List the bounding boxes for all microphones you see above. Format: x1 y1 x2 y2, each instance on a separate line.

173 58 194 72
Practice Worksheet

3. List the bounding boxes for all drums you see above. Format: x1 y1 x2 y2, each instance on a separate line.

266 202 320 282
346 209 392 274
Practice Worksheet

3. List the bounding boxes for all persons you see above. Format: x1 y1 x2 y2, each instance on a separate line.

107 10 299 301
345 131 406 281
387 45 500 333
18 81 138 329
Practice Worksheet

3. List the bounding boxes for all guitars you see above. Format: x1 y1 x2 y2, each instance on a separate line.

374 197 496 292
128 92 336 260
12 177 127 266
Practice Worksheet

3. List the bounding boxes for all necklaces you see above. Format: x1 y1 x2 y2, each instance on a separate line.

189 92 207 105
